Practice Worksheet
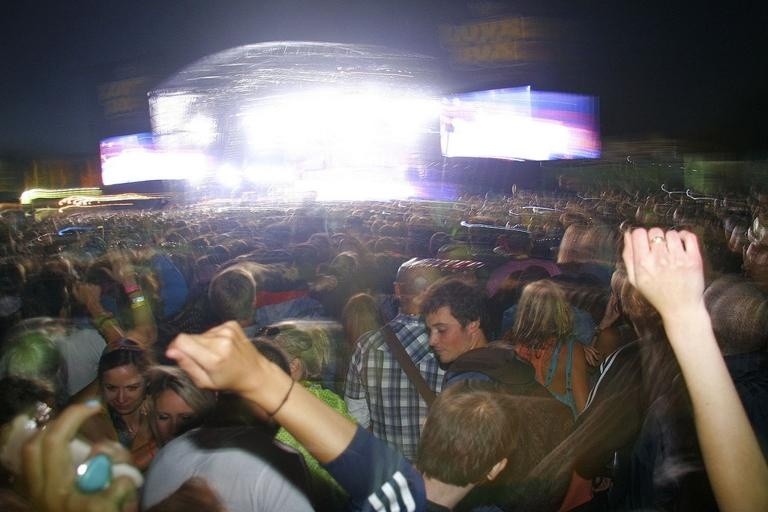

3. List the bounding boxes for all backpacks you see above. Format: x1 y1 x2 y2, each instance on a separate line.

491 378 572 512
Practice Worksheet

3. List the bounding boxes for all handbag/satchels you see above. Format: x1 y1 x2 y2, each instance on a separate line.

542 335 611 477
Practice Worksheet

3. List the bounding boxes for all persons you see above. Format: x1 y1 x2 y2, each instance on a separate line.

0 154 767 512
